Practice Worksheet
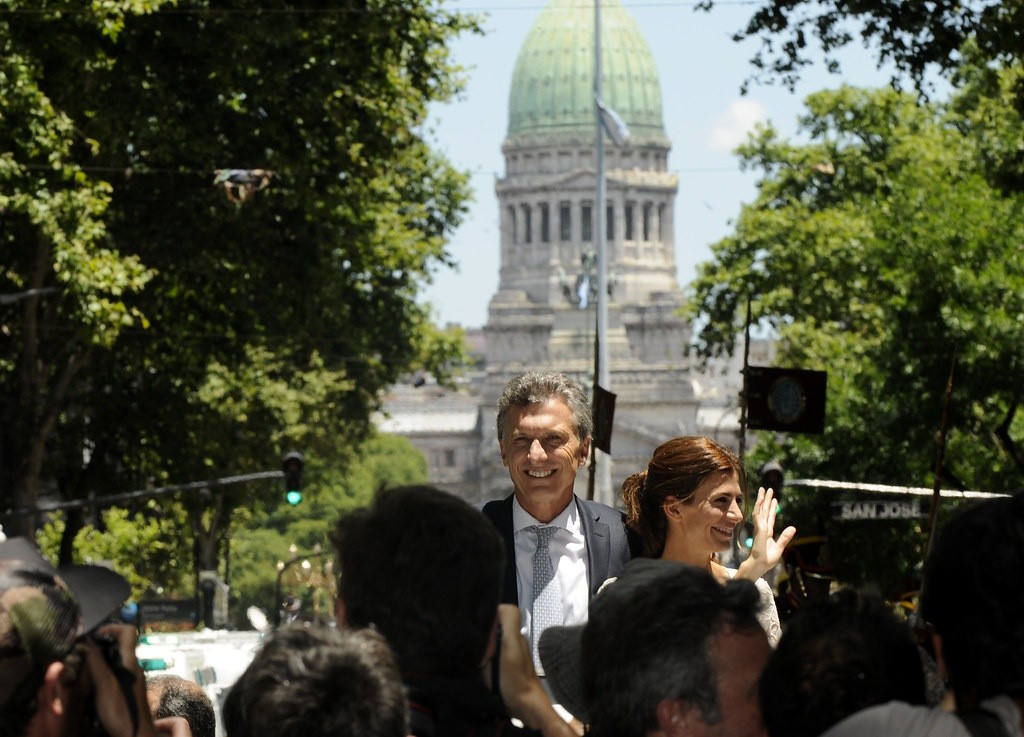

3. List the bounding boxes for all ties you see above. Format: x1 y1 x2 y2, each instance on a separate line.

525 524 563 677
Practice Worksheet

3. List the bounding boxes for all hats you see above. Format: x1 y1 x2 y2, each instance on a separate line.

0 537 132 706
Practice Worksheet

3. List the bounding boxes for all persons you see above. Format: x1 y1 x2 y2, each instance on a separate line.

478 372 646 737
598 435 796 649
0 483 1024 737
